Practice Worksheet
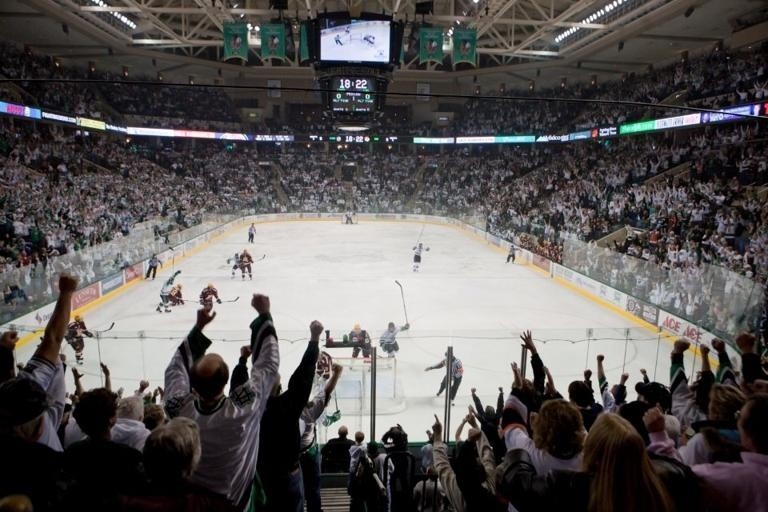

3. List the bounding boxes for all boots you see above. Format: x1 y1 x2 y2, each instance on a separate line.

165 308 171 312
155 307 162 312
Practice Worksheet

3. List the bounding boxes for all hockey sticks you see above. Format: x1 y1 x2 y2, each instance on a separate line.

82 323 115 336
203 297 239 302
249 254 266 265
394 280 409 325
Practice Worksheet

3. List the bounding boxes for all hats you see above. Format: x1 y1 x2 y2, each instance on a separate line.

1 377 49 427
635 381 671 411
71 386 116 421
